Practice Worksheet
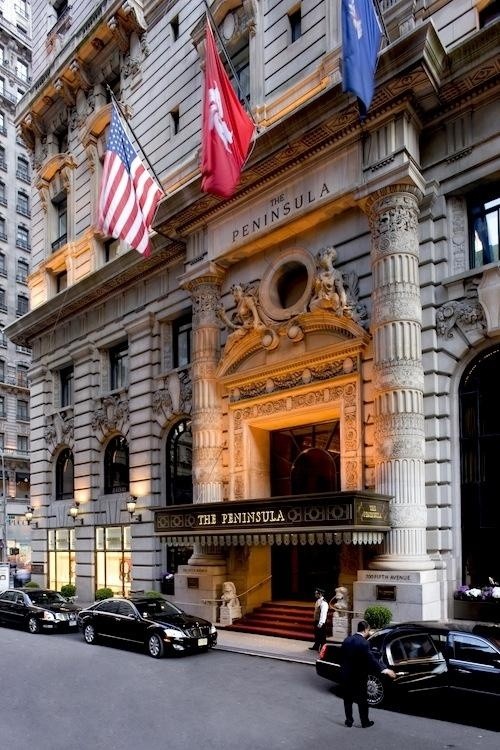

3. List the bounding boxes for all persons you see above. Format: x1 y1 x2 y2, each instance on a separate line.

214 284 262 356
340 621 396 728
309 588 329 653
308 245 360 324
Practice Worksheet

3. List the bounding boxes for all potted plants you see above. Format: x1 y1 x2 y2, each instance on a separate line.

94 588 114 605
365 606 392 637
60 585 78 605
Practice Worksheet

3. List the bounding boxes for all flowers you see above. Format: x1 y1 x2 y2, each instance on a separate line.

161 573 175 583
454 577 500 602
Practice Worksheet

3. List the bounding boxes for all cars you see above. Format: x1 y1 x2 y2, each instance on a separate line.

0 588 84 634
315 619 500 720
76 597 218 658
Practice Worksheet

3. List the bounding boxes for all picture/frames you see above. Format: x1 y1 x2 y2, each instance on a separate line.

376 585 396 601
187 577 199 588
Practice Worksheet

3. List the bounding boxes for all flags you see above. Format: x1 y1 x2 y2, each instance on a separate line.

199 11 257 199
341 0 384 123
98 100 165 260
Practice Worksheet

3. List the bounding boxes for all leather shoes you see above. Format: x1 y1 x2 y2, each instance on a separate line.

362 721 374 728
344 719 352 727
309 647 316 650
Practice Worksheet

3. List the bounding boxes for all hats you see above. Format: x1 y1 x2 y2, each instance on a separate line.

315 588 325 595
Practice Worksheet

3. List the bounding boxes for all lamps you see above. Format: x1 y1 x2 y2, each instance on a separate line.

24 506 38 529
69 500 84 526
126 495 142 523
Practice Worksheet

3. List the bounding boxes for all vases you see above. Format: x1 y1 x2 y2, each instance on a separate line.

161 583 174 596
454 600 500 621
